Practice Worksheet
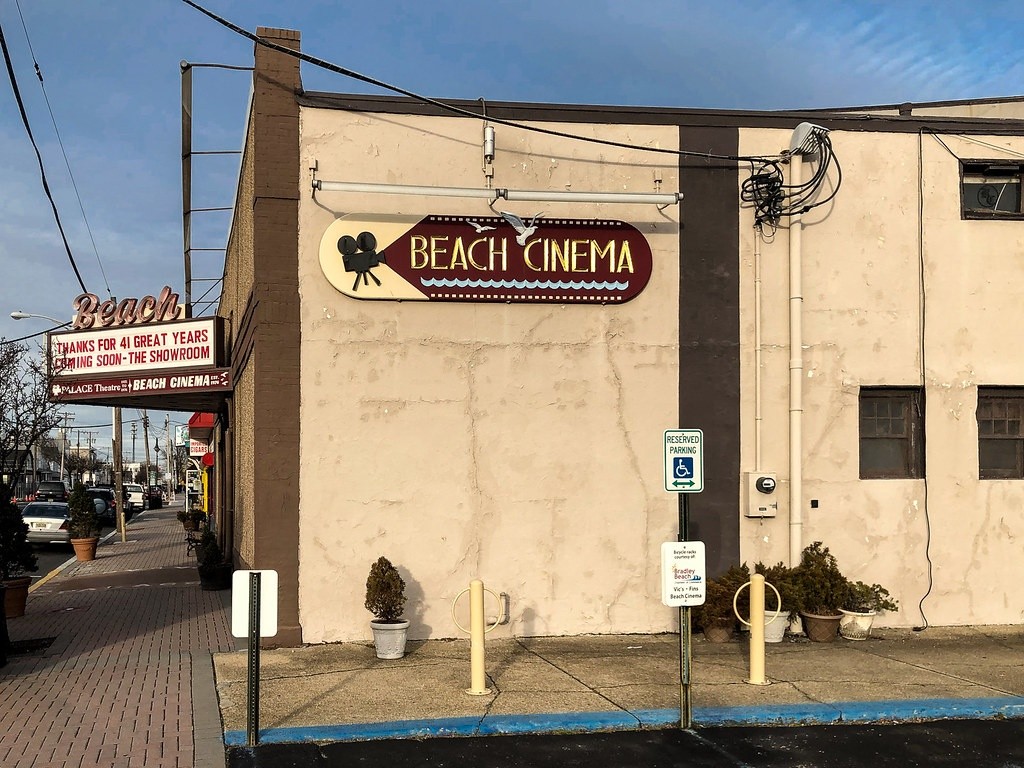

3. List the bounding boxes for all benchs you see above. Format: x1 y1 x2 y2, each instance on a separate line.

186 518 212 556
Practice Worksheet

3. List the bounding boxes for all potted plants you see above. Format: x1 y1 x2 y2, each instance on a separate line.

690 541 898 644
177 506 206 532
0 480 39 619
67 480 101 561
195 524 233 591
365 557 410 660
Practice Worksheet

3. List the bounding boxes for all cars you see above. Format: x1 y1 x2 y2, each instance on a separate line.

145 487 164 499
19 501 71 544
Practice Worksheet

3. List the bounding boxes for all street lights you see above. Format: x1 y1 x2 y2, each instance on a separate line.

10 311 125 532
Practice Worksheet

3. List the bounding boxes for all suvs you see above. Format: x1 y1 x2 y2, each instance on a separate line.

124 484 146 510
89 486 131 523
35 479 72 504
